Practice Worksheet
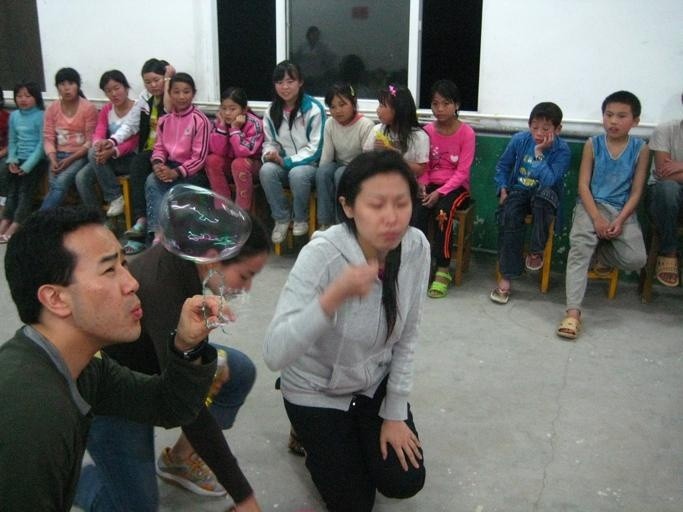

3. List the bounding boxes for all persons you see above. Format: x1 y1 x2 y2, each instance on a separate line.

257 59 326 245
362 83 429 179
261 148 432 512
202 86 264 212
410 78 476 300
39 66 98 215
558 91 647 339
648 94 683 288
0 79 46 246
144 72 211 249
1 94 10 221
121 58 176 257
74 70 142 222
490 101 572 306
315 82 375 229
292 26 340 79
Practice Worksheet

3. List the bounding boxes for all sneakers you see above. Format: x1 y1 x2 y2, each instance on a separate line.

272 224 289 243
107 195 124 216
293 222 308 236
156 447 227 497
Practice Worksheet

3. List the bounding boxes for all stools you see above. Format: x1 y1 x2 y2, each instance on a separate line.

427 199 476 290
34 169 51 200
274 188 316 258
100 175 137 231
585 246 620 302
45 164 77 204
494 213 555 294
640 208 683 304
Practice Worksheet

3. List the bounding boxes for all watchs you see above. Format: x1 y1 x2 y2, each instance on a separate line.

166 336 210 365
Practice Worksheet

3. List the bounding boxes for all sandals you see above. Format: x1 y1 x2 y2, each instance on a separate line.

429 272 451 298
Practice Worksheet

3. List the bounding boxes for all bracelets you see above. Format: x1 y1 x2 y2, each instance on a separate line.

163 75 172 83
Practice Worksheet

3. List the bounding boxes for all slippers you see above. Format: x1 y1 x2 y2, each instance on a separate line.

124 239 145 254
125 224 145 236
0 234 11 243
655 255 678 286
491 285 511 304
557 317 581 338
594 264 612 275
526 253 543 269
